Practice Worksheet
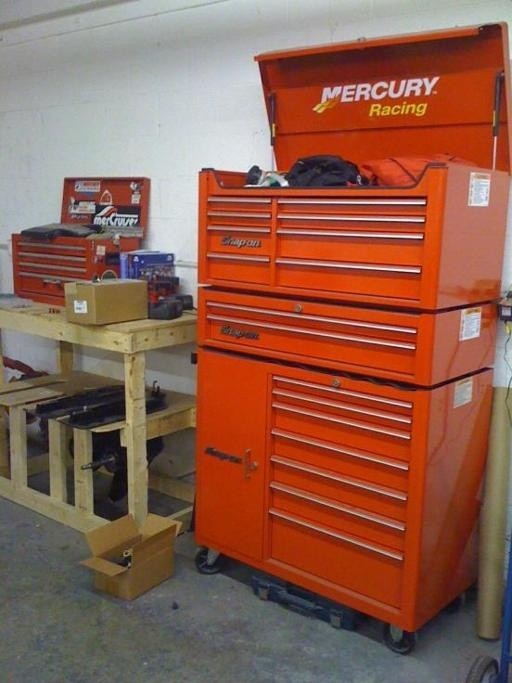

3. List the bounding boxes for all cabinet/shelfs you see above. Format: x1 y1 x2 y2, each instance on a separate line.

0 301 197 533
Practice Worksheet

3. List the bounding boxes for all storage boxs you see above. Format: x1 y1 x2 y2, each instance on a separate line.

64 279 147 325
79 513 183 601
